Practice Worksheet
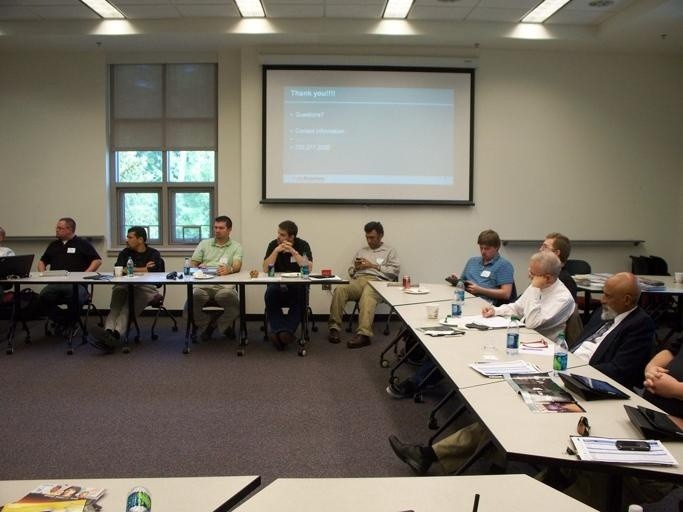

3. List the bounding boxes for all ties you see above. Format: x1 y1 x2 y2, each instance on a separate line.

583 318 613 343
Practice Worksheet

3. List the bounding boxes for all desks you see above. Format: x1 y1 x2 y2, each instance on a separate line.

227 472 604 510
568 274 683 353
427 365 683 485
0 468 261 512
382 297 586 441
370 280 471 360
0 265 345 358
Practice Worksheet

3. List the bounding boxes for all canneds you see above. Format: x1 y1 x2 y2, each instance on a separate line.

403 277 410 288
268 264 275 277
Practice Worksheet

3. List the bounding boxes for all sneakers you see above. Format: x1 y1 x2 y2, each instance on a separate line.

386 381 415 399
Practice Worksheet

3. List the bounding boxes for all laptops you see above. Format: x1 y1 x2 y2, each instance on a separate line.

0 254 35 280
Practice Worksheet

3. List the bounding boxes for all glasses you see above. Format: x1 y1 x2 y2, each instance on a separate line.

519 339 547 348
577 416 590 437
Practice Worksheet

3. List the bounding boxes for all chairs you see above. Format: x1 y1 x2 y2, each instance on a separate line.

345 272 400 338
560 254 679 347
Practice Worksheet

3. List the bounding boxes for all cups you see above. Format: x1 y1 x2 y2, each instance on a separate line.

425 303 438 319
113 267 123 278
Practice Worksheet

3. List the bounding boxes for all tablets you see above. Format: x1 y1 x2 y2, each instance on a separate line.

570 373 631 400
637 405 683 435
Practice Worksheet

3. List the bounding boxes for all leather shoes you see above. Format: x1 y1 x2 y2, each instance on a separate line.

386 433 433 476
277 330 293 345
328 329 340 343
201 323 215 342
225 325 235 340
270 333 283 351
347 333 370 348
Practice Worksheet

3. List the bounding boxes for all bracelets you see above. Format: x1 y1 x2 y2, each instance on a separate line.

230 266 234 274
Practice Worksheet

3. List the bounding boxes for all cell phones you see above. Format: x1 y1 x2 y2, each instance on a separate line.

616 440 651 452
356 258 362 264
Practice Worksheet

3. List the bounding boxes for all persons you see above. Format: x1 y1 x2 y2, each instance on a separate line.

60 487 80 499
263 221 312 350
183 216 243 341
329 222 400 348
38 218 102 335
385 231 578 398
0 226 15 303
389 272 655 490
531 332 681 510
86 226 159 353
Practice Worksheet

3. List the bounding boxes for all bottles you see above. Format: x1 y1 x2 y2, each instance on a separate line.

553 332 568 377
183 257 190 278
507 315 519 353
126 256 133 277
300 252 308 279
451 290 462 321
455 278 464 305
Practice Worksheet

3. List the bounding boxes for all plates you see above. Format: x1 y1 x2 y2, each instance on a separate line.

402 287 430 294
193 274 212 279
